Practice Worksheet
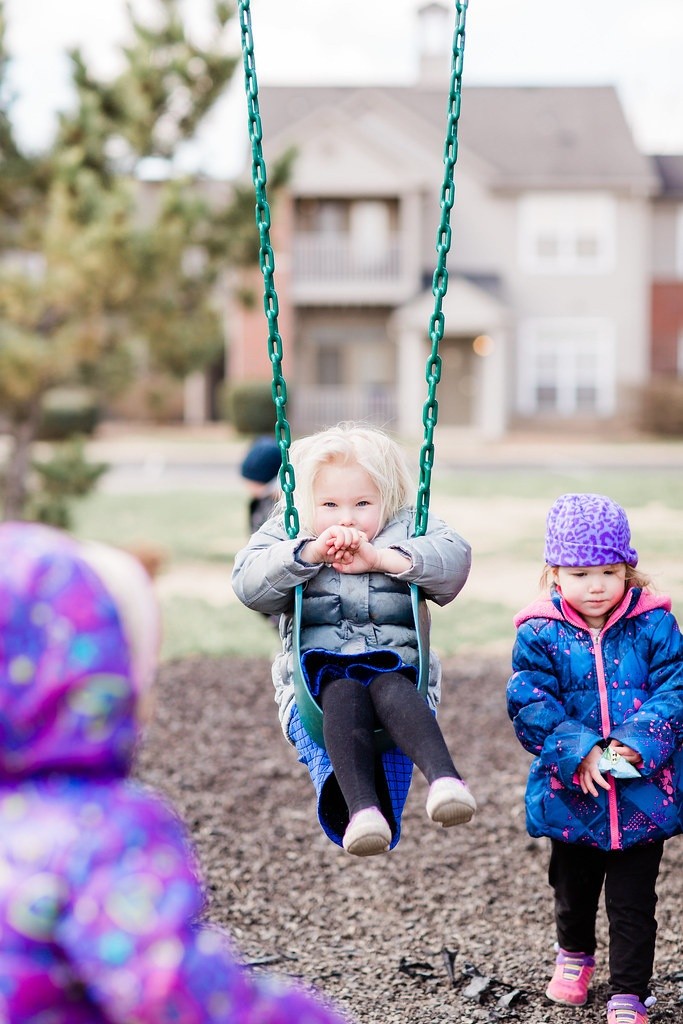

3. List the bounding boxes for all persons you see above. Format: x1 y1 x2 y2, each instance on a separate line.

229 419 475 854
0 514 348 1024
238 437 283 537
503 496 683 1024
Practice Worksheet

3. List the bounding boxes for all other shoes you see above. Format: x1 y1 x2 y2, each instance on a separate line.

427 779 476 828
545 948 597 1006
340 808 393 855
606 994 649 1023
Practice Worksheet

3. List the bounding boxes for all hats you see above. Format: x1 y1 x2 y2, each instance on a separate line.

240 437 286 484
545 492 636 571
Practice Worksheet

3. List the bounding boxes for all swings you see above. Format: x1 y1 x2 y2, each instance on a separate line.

236 0 468 750
